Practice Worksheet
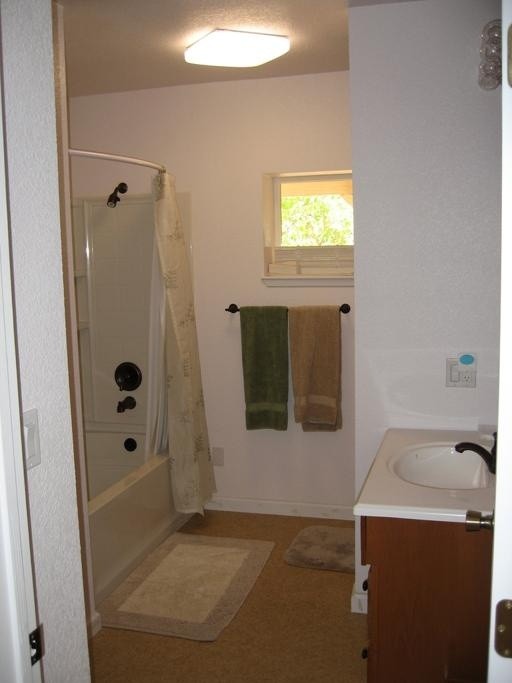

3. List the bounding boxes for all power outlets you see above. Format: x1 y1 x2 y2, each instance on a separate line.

445 357 477 388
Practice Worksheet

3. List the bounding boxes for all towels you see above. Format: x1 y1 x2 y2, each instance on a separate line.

240 305 342 432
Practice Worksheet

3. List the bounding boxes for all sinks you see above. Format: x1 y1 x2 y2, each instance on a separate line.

391 440 493 491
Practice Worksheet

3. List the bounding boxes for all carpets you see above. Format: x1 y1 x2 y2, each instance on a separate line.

94 531 275 642
283 526 355 573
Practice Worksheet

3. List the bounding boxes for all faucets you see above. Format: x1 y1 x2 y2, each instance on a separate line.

454 430 497 476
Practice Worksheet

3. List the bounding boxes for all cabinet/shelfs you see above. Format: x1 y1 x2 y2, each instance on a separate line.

361 516 493 683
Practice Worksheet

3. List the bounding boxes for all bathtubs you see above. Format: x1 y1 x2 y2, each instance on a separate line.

85 428 196 605
